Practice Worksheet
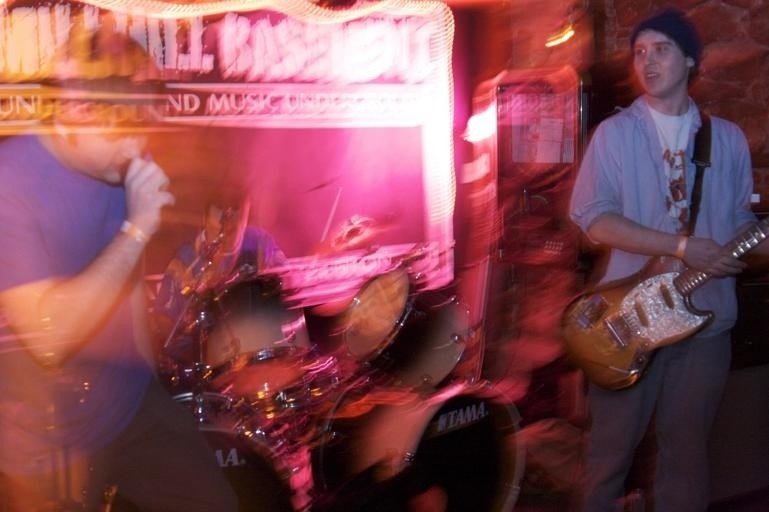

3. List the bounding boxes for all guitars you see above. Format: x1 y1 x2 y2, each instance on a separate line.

560 218 768 390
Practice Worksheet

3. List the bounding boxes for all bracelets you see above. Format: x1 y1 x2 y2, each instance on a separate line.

673 233 689 261
122 219 150 245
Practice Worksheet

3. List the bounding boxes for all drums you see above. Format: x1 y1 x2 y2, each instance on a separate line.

324 369 526 511
198 271 316 401
112 392 293 509
344 269 471 393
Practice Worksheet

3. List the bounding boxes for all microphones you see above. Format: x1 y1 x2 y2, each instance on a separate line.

185 232 224 278
209 260 260 300
219 208 238 237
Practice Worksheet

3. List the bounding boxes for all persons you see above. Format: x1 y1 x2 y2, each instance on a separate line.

151 182 291 365
566 9 767 511
2 30 239 511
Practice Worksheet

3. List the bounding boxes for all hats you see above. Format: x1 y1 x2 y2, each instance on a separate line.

631 9 702 66
